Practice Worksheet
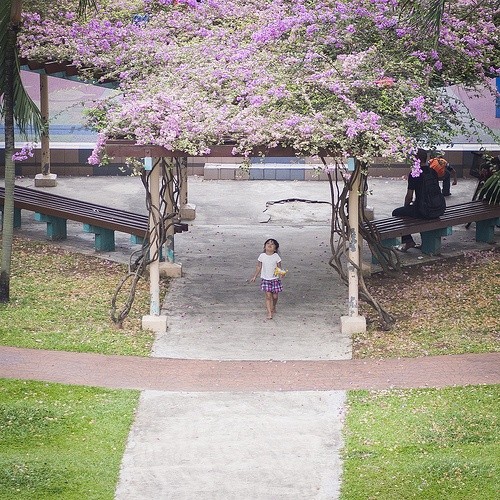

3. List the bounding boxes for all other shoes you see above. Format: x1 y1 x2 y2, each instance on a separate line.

398 240 416 252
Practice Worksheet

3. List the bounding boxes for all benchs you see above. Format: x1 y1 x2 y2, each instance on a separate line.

361 201 500 265
0 185 188 260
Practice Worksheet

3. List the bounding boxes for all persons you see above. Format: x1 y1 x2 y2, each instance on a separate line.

427 154 457 196
250 239 282 320
392 149 446 252
